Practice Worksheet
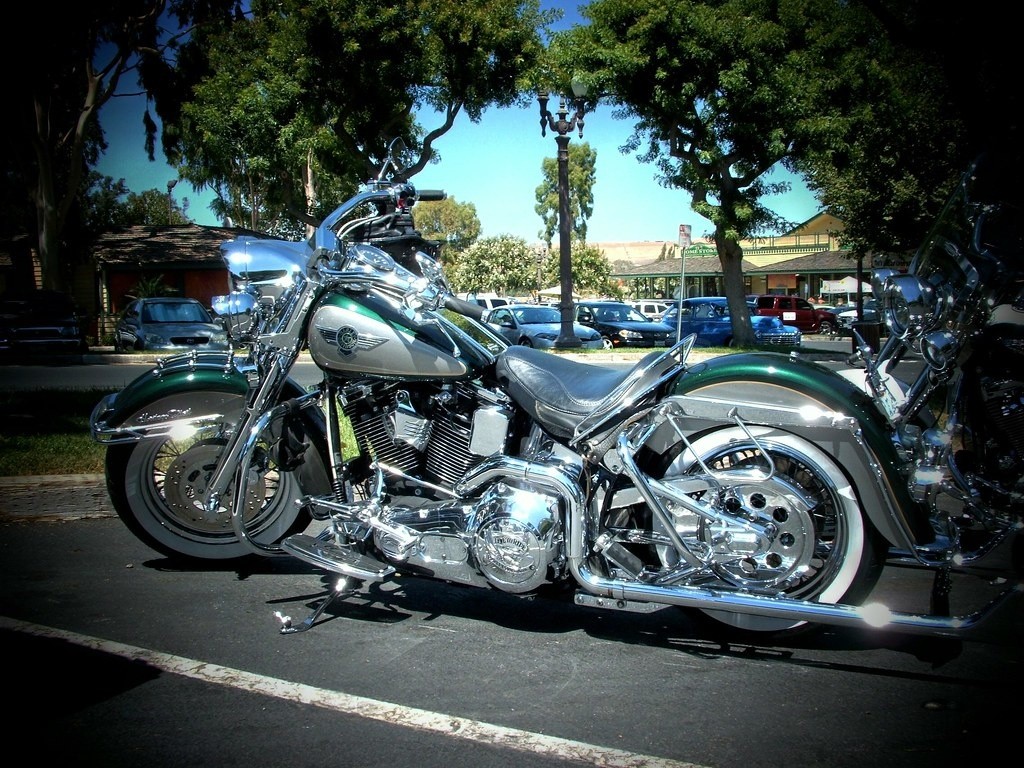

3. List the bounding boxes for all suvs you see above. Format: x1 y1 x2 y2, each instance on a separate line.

838 298 884 331
744 293 842 336
466 298 526 322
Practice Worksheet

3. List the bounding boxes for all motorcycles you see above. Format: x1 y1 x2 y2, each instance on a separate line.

90 131 1023 642
788 160 1022 572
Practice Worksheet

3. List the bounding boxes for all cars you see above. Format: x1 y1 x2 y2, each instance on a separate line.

484 304 605 355
114 295 230 355
0 299 85 359
617 298 681 324
553 300 678 348
658 296 803 355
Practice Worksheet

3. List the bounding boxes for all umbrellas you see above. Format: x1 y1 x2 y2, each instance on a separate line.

536 286 582 303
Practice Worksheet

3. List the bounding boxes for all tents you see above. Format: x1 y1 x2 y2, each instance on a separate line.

820 276 873 304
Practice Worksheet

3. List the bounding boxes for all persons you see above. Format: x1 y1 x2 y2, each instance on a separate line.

653 289 664 298
791 292 873 307
